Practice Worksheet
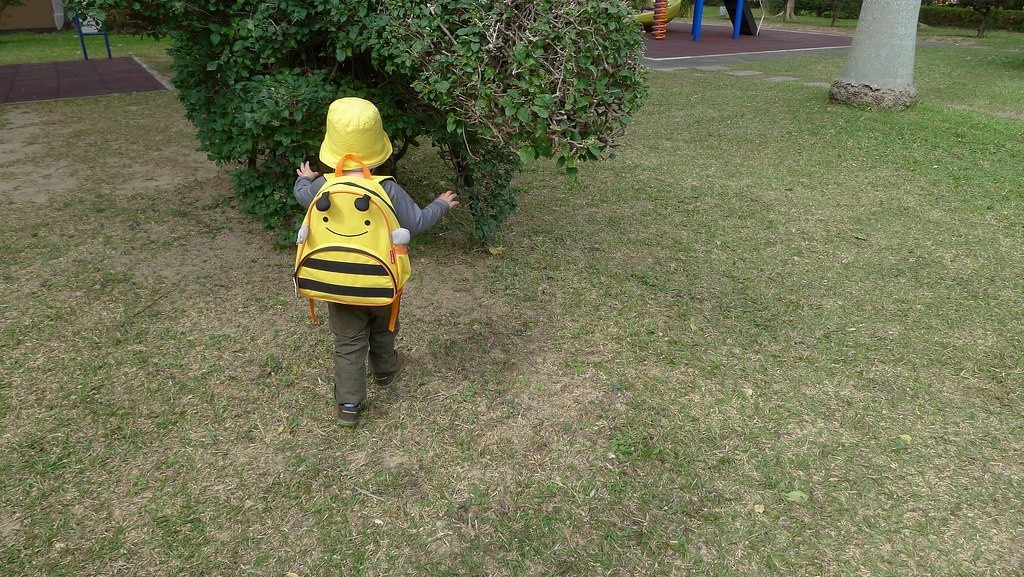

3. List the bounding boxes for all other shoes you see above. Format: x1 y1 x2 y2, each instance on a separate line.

374 349 406 389
337 403 361 426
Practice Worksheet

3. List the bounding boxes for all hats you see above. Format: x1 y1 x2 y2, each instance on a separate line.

319 97 393 170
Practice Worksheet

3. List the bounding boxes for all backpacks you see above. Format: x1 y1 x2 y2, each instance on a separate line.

293 154 412 307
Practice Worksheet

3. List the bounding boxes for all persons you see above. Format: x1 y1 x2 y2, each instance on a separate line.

294 97 460 426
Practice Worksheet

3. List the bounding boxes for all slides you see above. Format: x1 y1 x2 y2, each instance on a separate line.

627 0 682 27
